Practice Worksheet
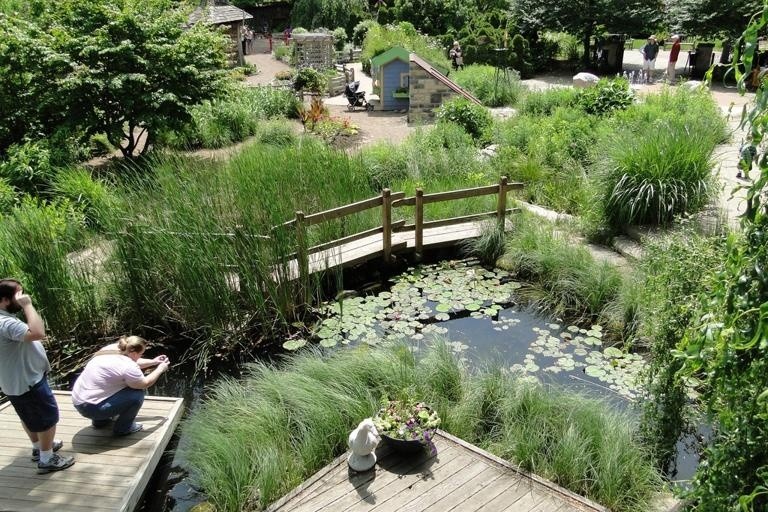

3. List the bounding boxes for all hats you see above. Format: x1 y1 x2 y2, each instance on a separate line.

671 34 680 40
649 35 656 40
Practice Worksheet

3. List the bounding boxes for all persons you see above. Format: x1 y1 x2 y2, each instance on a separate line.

240 25 258 56
71 333 171 436
449 39 465 71
641 35 660 83
666 35 681 86
0 279 77 475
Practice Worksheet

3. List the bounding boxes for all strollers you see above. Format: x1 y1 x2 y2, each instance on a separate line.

342 81 374 113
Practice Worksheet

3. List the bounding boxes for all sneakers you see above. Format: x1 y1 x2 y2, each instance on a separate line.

657 79 665 83
37 454 75 474
123 422 143 434
31 440 62 460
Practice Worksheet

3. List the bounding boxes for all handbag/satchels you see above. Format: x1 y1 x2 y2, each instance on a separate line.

456 57 463 65
